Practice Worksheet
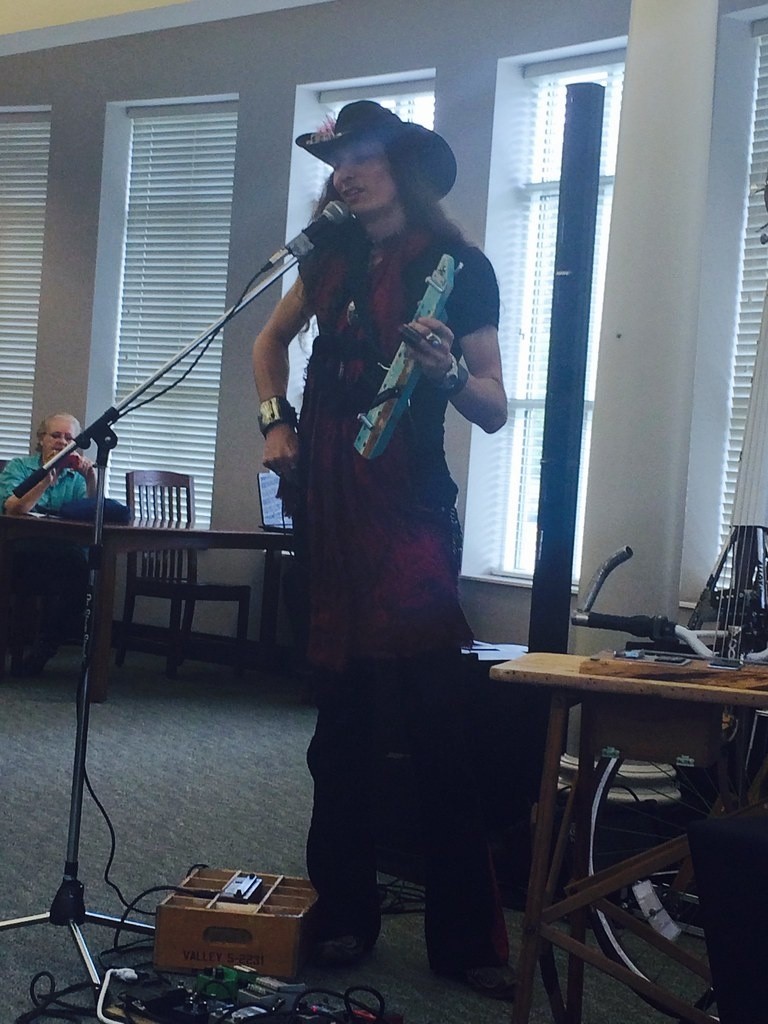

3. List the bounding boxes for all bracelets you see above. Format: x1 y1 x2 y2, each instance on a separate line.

445 363 469 396
256 396 299 435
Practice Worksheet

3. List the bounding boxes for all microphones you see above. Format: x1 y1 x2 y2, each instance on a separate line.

260 200 351 274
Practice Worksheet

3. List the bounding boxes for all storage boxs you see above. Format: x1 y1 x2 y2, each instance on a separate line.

154 866 321 979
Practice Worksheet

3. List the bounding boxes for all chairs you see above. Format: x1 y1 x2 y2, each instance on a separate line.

116 470 252 677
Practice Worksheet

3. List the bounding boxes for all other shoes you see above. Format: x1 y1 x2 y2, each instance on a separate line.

10 642 24 679
467 968 520 1000
304 933 365 963
24 650 58 679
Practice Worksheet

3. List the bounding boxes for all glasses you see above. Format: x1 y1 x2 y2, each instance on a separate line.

43 431 77 441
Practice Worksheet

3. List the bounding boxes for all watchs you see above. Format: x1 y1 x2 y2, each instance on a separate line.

430 353 458 390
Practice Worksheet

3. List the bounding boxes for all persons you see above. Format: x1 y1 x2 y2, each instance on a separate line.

0 412 109 675
248 103 515 998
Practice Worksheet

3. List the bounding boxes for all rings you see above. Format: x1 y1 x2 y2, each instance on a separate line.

291 465 296 470
84 463 87 467
426 333 443 345
277 472 282 475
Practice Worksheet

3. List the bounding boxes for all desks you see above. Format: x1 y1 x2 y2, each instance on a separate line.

490 652 768 1024
0 516 293 704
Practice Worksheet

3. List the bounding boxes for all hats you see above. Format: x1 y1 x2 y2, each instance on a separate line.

296 101 456 200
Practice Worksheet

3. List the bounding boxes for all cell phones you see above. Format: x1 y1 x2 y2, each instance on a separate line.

655 657 685 663
710 659 741 667
55 455 79 469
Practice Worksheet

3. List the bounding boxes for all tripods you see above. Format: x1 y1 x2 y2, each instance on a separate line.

0 234 315 1009
